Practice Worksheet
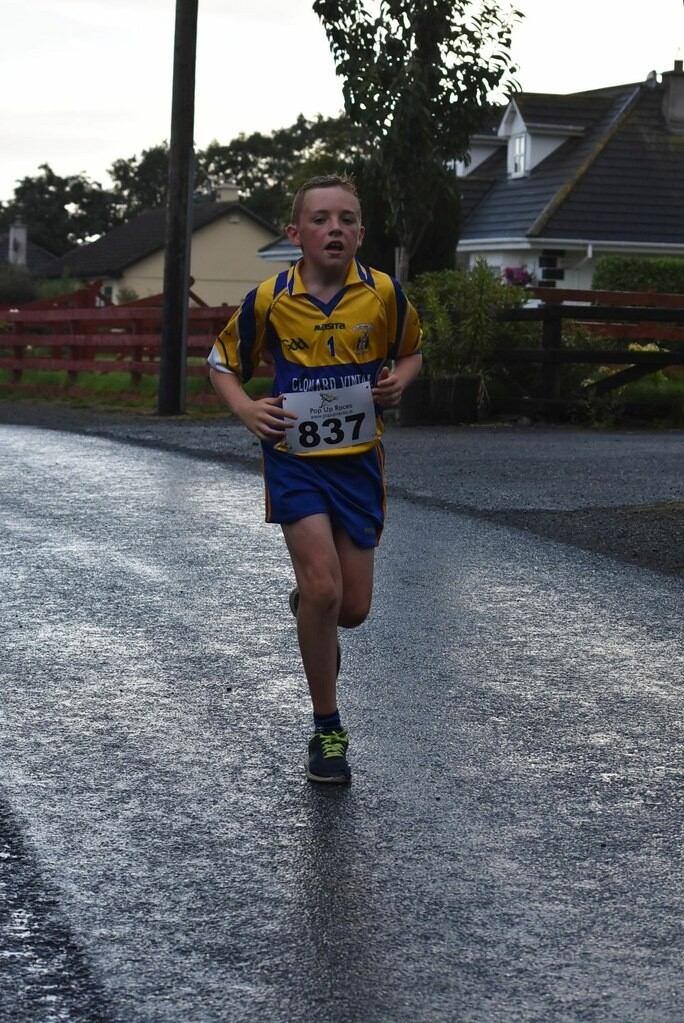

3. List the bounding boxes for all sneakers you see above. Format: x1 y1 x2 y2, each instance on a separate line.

303 729 352 784
289 587 341 679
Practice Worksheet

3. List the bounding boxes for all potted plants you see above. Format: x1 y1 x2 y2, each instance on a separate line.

392 252 498 425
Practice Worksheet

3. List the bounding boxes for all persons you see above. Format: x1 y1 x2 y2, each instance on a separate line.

206 174 422 785
